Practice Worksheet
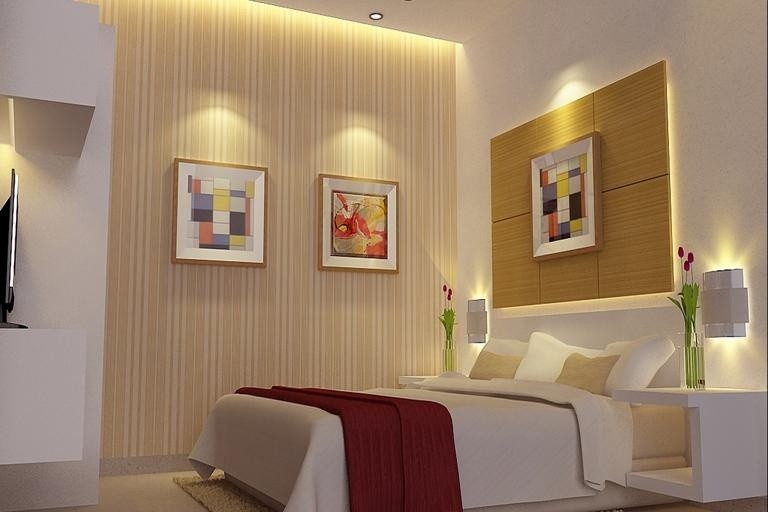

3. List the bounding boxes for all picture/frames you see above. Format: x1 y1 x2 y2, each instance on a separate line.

171 158 269 268
529 131 605 263
317 173 400 273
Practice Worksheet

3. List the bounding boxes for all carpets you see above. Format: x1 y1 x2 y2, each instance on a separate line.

172 472 273 512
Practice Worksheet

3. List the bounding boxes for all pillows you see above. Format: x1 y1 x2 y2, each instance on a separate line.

469 330 674 402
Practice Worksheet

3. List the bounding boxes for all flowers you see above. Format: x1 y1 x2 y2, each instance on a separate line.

436 283 458 370
667 245 705 385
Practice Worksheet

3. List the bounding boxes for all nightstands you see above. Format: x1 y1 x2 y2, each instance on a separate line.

610 386 767 506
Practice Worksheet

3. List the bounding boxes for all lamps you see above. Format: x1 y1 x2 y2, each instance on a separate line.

466 298 490 343
702 267 750 338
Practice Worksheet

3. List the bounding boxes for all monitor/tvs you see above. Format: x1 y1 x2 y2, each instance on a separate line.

0 168 28 328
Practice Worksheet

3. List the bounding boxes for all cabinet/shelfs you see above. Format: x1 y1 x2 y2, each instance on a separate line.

0 327 89 464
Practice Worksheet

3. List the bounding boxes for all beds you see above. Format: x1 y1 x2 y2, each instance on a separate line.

190 302 691 511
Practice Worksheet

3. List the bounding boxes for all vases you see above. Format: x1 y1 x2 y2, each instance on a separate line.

440 337 458 373
676 332 708 392
